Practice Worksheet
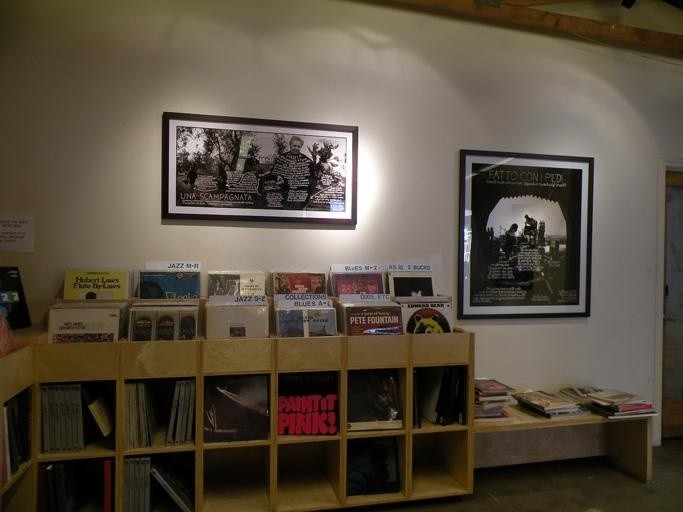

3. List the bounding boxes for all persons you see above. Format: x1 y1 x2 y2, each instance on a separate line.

503 223 519 245
524 214 537 246
272 136 313 206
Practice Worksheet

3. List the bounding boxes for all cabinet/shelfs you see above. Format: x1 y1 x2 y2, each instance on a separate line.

0 326 475 512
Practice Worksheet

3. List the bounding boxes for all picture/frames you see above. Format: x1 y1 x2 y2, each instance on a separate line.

160 110 359 227
457 147 595 319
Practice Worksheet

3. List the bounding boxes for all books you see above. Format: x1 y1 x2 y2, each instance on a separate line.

207 268 337 338
0 265 32 330
200 367 466 496
473 376 658 422
37 379 196 511
330 263 453 336
1 388 33 491
46 263 201 342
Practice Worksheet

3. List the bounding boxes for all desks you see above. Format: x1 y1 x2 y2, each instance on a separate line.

472 403 653 484
